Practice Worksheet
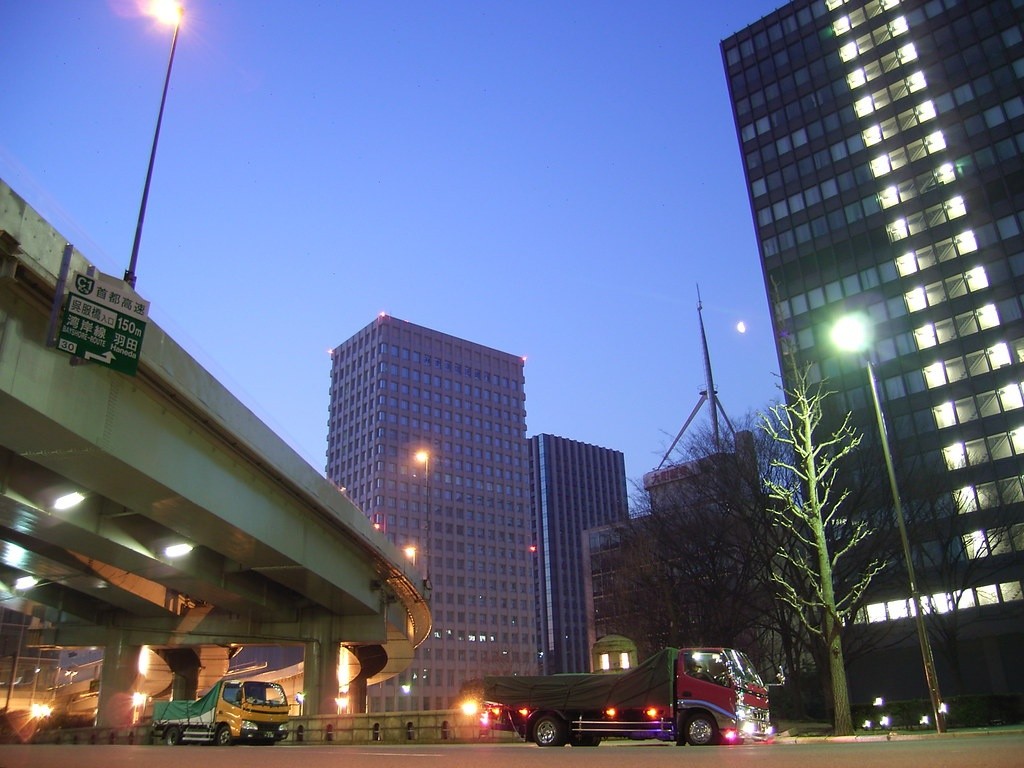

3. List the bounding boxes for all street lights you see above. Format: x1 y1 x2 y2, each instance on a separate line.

829 309 952 728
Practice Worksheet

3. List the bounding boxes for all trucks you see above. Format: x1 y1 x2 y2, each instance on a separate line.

479 644 786 746
150 679 301 747
